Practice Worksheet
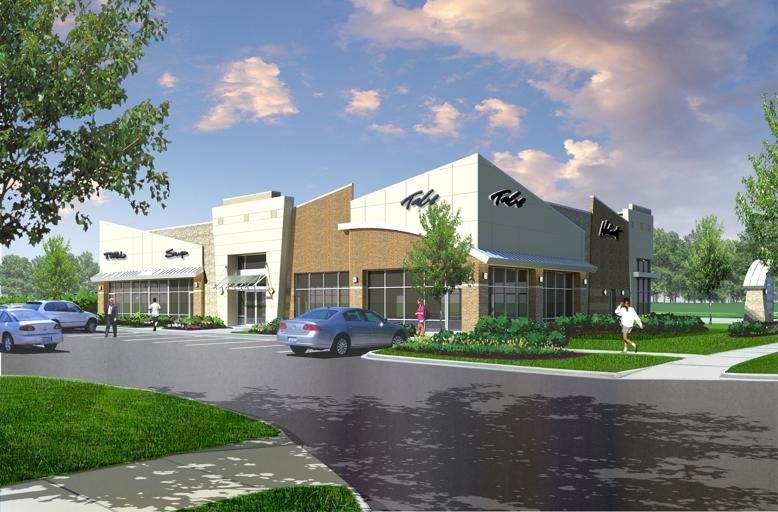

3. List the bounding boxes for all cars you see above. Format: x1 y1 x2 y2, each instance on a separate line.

276 307 410 358
0 299 98 353
